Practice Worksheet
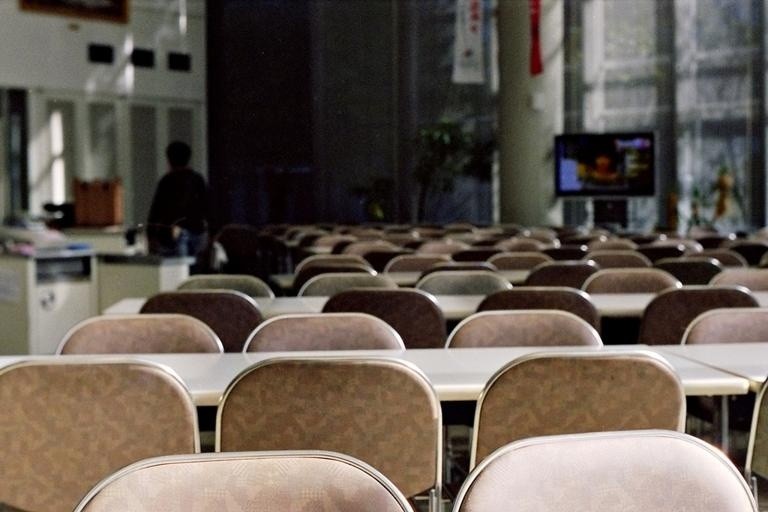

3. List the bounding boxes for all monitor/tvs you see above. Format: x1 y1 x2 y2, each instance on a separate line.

554 131 656 197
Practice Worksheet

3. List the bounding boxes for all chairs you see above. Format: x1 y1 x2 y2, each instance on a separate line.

3 216 768 510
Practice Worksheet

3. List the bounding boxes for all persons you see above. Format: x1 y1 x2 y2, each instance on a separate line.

147 140 209 255
587 148 617 177
